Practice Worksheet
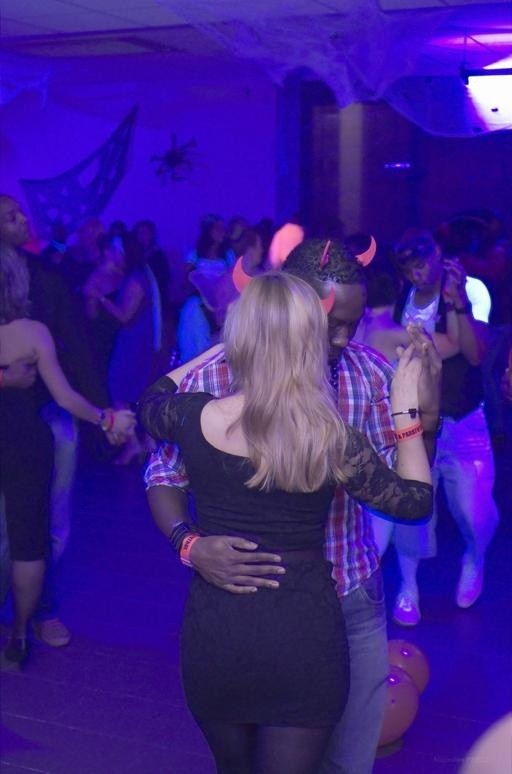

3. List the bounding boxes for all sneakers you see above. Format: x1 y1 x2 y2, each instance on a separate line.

457 556 487 609
392 587 421 628
34 616 73 645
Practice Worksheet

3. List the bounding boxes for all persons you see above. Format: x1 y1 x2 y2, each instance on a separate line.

0 195 512 661
134 239 443 774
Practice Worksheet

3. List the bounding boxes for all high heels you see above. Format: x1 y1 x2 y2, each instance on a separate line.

7 638 30 663
